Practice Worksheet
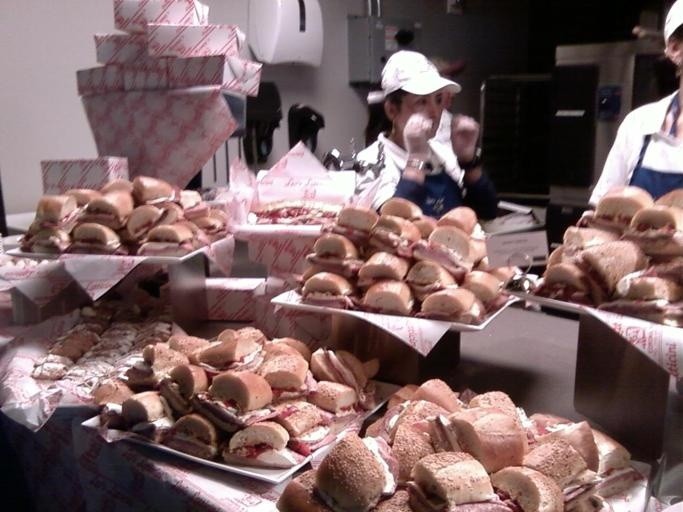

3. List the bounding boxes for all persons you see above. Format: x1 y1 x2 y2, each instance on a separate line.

427 55 468 151
355 46 500 227
579 0 683 210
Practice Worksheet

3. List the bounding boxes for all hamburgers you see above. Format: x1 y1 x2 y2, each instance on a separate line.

20 176 231 255
276 384 640 512
96 327 383 470
533 183 682 323
299 197 513 324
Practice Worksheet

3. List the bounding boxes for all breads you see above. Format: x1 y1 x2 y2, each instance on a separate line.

31 303 174 384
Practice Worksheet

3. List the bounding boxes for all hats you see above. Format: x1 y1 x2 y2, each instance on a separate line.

661 0 683 42
365 50 461 104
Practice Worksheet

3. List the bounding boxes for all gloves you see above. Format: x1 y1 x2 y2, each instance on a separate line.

402 114 433 164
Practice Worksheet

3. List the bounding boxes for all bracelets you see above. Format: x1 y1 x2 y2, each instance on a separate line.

451 147 484 172
405 154 435 176
